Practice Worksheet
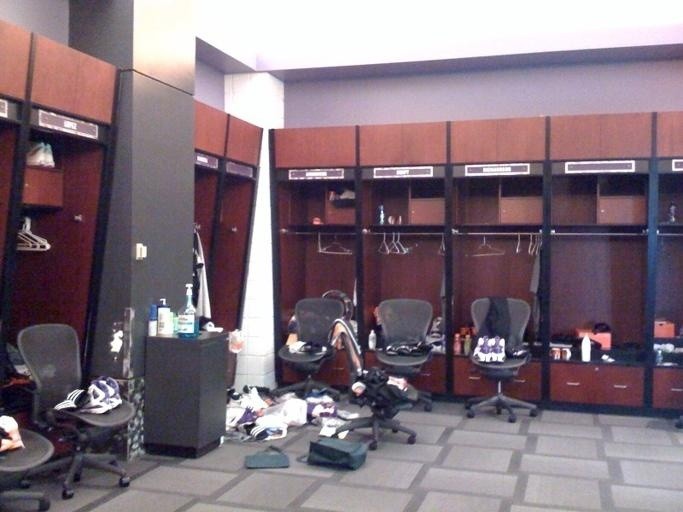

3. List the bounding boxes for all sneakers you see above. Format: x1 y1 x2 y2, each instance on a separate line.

230 387 268 410
548 348 572 360
80 377 121 416
473 336 505 365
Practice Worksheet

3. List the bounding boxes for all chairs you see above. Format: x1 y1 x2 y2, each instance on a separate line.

0 408 56 512
368 297 434 411
17 323 137 500
268 298 344 404
329 318 419 450
465 298 539 422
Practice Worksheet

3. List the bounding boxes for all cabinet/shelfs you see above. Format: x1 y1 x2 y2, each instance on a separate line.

545 112 654 408
649 111 683 411
449 116 545 403
0 19 121 456
269 125 358 389
358 122 449 398
143 332 228 458
193 102 263 406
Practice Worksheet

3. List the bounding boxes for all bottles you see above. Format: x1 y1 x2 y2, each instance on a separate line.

655 349 664 367
147 304 158 336
376 205 386 225
668 203 678 224
452 327 506 364
369 330 377 351
581 333 591 362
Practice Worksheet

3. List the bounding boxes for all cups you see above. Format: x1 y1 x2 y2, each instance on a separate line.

562 349 572 360
312 217 321 225
549 347 560 360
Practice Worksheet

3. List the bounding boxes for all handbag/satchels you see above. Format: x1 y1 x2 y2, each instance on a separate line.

297 435 367 471
245 445 288 469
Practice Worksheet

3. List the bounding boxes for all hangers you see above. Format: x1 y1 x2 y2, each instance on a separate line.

16 217 52 253
465 233 508 258
515 233 543 254
316 232 352 255
437 231 447 257
377 229 409 257
